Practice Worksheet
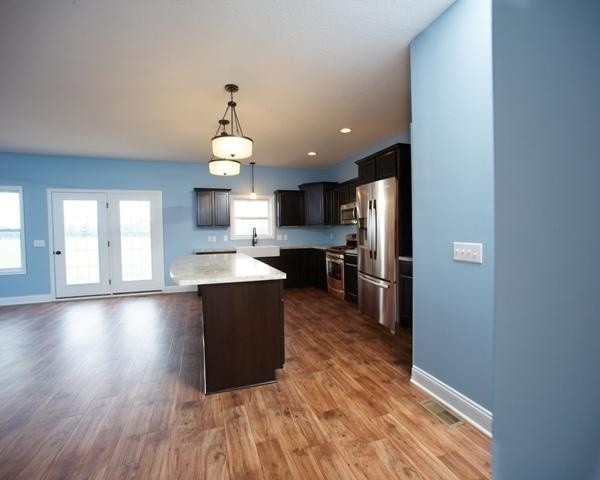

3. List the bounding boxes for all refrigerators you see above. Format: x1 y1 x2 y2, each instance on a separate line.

355 177 399 331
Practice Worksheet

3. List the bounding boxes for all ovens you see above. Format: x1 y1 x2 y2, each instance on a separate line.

325 251 345 301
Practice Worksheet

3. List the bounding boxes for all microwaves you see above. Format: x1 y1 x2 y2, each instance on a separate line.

340 201 357 226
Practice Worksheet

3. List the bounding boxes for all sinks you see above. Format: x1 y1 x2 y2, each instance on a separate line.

235 246 281 258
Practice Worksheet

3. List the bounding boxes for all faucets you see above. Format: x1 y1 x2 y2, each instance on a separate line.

252 227 258 246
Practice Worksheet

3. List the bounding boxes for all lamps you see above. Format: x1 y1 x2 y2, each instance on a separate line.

208 120 241 176
211 83 253 160
249 162 258 199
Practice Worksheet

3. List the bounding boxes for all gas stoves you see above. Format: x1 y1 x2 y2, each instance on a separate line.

326 245 356 254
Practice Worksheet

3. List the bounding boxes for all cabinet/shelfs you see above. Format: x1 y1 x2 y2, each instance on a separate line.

193 187 232 228
354 143 411 185
280 249 305 288
334 178 358 204
305 248 326 292
300 182 337 226
274 190 305 226
